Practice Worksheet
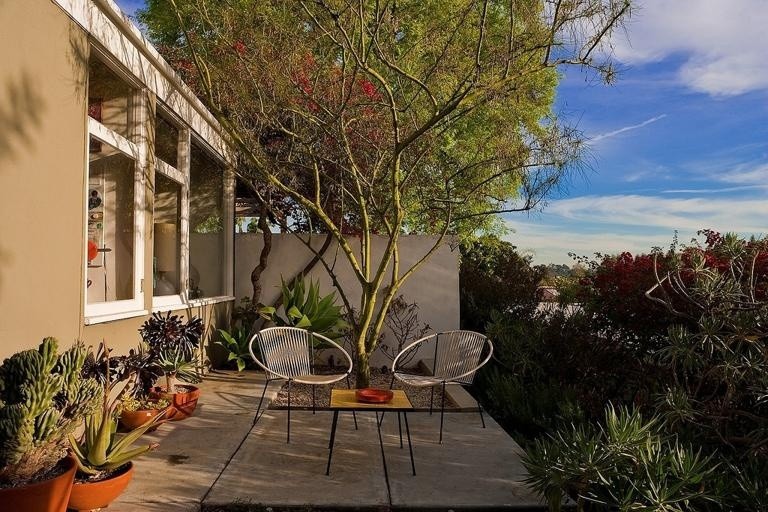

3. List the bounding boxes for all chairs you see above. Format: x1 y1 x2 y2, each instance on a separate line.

249 327 358 444
378 329 494 445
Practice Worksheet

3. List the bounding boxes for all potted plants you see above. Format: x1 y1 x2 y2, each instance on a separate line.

117 348 172 433
0 337 104 512
68 340 173 511
138 310 200 421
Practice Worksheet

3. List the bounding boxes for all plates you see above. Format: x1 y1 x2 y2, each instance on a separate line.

355 388 394 404
